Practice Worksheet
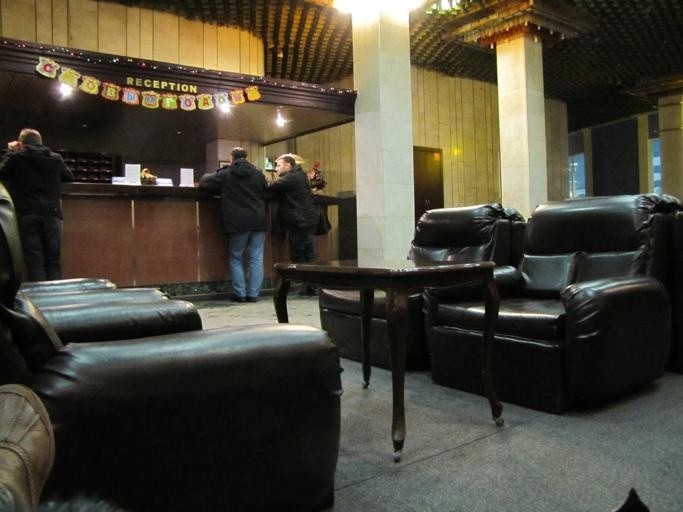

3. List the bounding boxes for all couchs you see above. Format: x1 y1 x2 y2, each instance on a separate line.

421 193 673 413
0 184 342 510
320 203 511 375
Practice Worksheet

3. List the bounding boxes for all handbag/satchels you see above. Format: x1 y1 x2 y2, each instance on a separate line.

304 173 332 236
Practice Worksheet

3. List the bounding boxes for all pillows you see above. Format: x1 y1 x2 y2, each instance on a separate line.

519 250 587 295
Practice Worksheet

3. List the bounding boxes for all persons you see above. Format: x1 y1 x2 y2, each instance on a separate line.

199 146 268 302
0 128 72 282
267 155 320 297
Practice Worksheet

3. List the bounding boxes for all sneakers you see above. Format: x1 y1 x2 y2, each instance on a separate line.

246 296 258 303
230 294 246 303
298 286 319 297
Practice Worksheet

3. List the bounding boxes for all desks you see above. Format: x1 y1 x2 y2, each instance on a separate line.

58 182 340 302
